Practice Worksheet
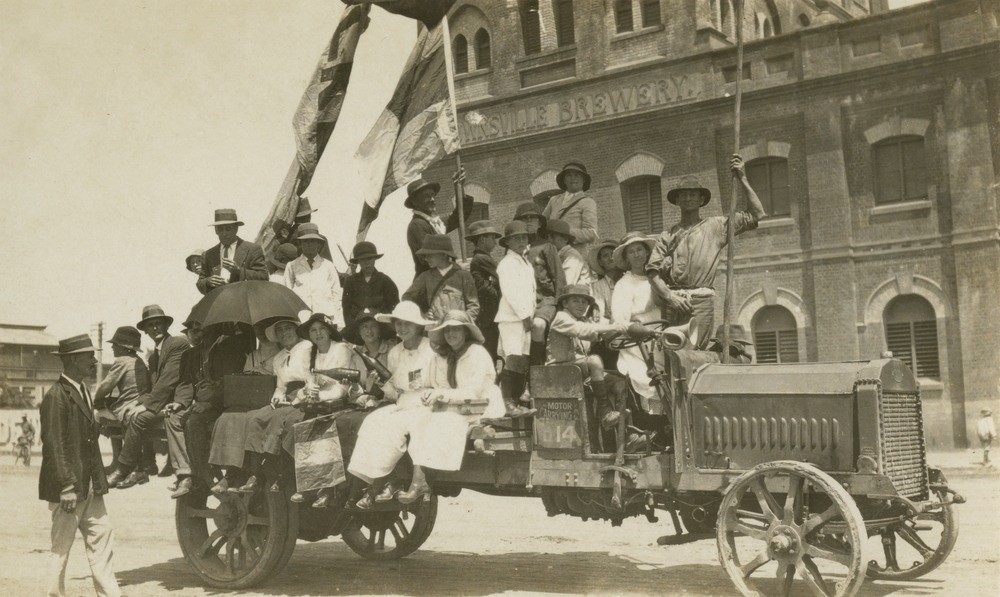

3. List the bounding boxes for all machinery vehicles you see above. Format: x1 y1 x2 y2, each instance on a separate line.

176 319 967 593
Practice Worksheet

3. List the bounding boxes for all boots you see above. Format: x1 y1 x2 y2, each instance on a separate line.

591 380 619 430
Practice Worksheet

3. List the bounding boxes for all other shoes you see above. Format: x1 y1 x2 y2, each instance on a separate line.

105 463 404 509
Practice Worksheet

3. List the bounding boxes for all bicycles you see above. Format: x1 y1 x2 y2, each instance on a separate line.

14 433 36 468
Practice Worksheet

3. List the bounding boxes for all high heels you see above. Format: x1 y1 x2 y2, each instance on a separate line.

399 476 430 502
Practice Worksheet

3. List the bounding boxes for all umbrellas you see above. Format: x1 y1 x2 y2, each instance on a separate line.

182 279 313 366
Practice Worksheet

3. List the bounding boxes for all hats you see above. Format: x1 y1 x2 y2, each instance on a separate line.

556 158 591 191
464 201 576 247
136 305 173 330
558 284 595 305
415 234 458 260
271 243 298 268
266 300 486 347
350 242 383 263
186 250 206 271
106 326 143 353
297 223 324 241
296 197 318 217
209 209 244 226
587 231 657 275
405 179 441 208
667 175 711 207
50 334 103 354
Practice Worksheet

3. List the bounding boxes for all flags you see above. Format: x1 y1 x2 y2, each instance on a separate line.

254 0 371 275
352 23 462 244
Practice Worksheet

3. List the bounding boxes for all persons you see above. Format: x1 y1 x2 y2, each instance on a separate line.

13 153 766 597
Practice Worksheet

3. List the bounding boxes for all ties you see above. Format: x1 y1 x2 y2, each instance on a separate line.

80 384 91 416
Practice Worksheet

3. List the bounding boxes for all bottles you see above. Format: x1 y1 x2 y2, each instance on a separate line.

353 347 392 384
311 367 360 383
